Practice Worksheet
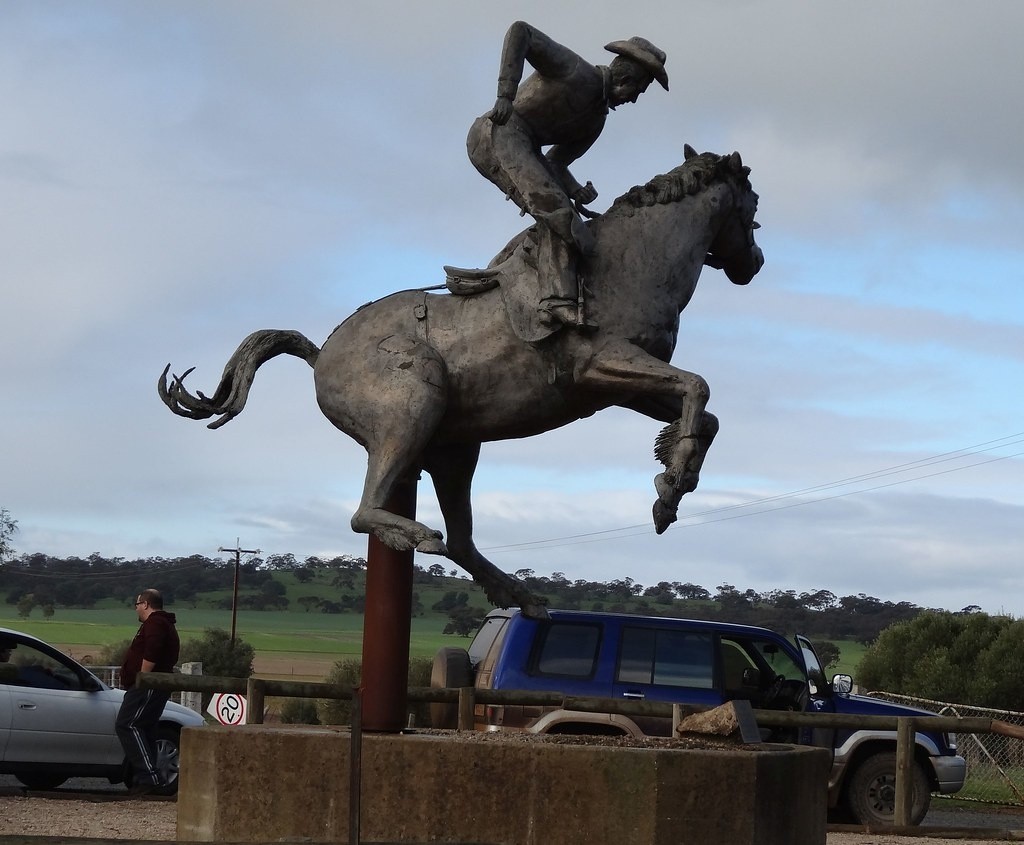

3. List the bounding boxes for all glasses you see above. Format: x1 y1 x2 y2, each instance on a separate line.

135 600 150 606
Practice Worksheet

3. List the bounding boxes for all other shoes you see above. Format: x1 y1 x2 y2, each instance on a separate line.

128 783 166 799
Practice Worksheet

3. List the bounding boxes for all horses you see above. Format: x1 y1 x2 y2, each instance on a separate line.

155 143 766 618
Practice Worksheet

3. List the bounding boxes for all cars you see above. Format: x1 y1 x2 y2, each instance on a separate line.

0 627 208 798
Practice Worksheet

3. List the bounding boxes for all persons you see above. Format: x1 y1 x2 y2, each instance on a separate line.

465 21 669 330
0 648 25 683
114 588 180 797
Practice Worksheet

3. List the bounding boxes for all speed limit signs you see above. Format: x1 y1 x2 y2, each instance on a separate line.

208 693 246 725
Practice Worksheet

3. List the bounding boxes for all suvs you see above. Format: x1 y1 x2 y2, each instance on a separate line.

429 604 966 838
